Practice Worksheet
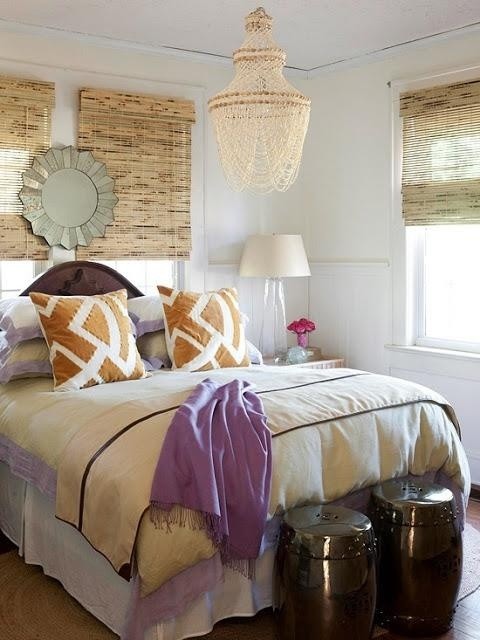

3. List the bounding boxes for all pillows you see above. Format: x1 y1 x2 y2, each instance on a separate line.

1 281 266 394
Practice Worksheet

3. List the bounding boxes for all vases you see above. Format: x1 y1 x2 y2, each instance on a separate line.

297 332 310 350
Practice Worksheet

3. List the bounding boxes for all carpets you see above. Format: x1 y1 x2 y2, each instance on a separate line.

1 523 479 640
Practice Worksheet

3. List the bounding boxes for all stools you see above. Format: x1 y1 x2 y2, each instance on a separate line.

269 473 466 640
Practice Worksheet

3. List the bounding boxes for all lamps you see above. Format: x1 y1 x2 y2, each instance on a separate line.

239 230 313 362
205 1 316 196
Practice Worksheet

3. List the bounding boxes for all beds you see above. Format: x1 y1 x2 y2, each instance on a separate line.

2 259 474 640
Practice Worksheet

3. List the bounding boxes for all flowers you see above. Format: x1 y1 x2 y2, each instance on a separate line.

287 316 318 334
